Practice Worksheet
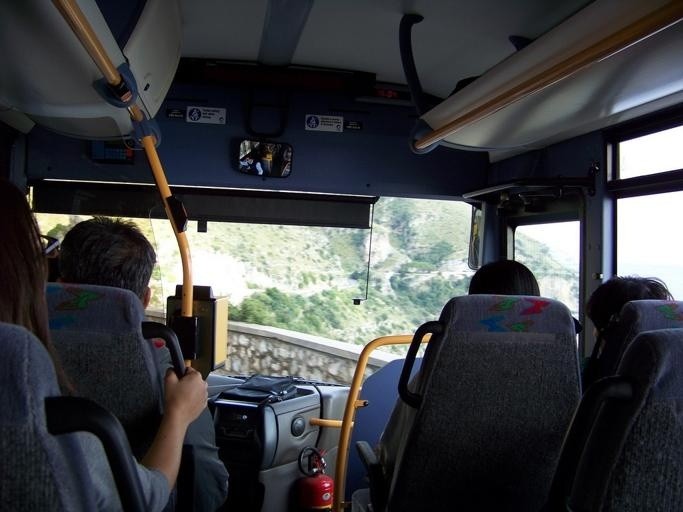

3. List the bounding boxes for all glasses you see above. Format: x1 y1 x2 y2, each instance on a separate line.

41 234 59 259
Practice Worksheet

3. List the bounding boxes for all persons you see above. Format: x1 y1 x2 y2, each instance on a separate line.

345 259 541 512
55 212 231 512
586 276 675 369
0 176 208 512
240 155 264 176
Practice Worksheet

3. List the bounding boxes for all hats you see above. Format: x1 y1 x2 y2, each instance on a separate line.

470 260 583 333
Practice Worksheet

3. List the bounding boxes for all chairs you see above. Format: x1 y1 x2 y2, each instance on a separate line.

1 283 196 512
352 294 681 512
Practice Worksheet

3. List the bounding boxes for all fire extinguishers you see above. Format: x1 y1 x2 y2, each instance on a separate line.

295 444 336 511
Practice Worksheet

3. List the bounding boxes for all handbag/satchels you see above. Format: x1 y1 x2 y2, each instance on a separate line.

221 374 296 402
